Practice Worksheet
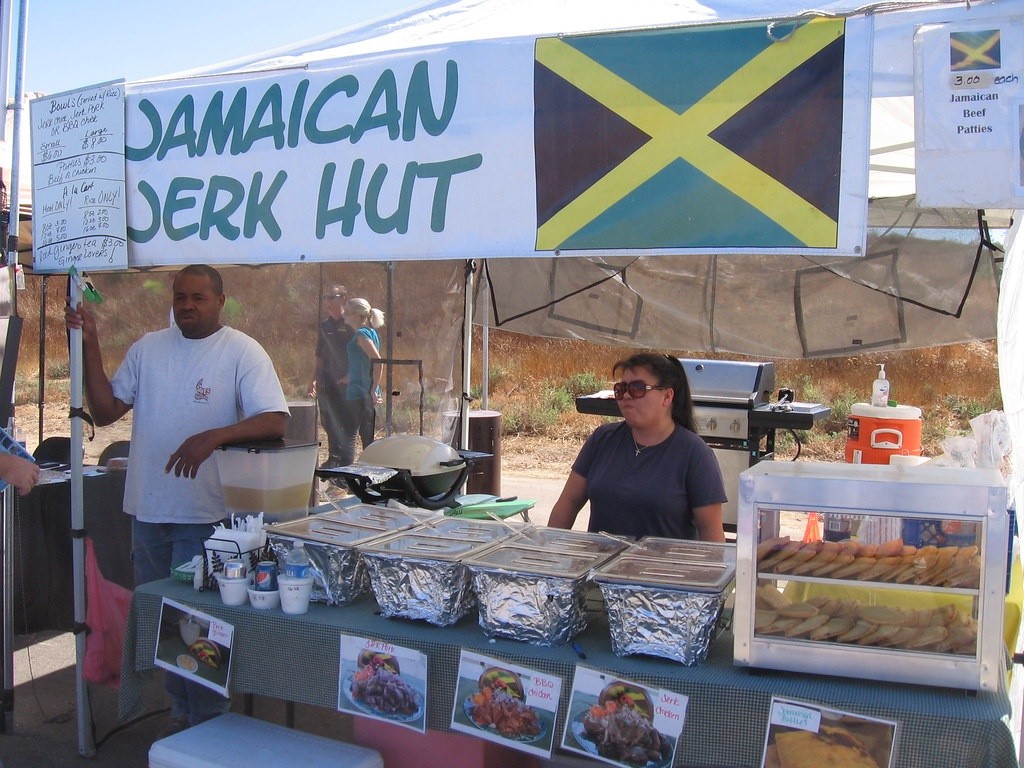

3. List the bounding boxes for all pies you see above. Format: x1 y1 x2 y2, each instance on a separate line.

754 535 980 656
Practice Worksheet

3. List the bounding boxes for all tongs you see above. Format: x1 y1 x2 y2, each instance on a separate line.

771 394 789 412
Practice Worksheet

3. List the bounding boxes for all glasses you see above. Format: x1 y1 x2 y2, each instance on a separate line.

613 380 669 403
323 294 342 300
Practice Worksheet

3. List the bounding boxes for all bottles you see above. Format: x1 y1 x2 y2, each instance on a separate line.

285 541 309 579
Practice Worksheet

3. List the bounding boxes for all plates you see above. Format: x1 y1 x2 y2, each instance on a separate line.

343 674 424 722
463 695 546 744
176 654 198 673
571 708 673 768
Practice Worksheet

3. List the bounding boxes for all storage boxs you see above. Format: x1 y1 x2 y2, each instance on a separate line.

146 708 387 768
844 402 923 466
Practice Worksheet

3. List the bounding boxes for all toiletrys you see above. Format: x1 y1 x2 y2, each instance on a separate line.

872 363 889 407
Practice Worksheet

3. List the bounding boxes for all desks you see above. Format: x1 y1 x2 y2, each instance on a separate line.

2 460 134 632
116 577 1019 768
441 491 535 525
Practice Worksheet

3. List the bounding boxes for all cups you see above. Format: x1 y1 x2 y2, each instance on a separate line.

277 574 314 615
179 621 199 646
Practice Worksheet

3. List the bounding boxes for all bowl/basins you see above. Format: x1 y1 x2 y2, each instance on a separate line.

247 584 280 610
217 577 249 605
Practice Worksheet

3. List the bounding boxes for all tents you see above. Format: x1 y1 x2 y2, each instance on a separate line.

69 0 1024 757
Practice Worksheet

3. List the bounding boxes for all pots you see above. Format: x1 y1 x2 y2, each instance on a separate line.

316 434 469 510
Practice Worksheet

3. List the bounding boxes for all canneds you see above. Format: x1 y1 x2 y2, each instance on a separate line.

223 559 247 580
254 561 278 592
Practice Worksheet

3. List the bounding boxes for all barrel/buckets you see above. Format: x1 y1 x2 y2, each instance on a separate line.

845 403 922 466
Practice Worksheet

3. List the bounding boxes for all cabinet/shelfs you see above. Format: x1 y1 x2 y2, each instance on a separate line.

733 456 1009 697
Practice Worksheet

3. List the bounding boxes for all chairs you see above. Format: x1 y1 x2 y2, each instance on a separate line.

33 436 87 464
98 438 131 465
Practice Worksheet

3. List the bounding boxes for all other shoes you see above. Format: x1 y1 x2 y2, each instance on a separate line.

156 716 192 740
320 455 347 469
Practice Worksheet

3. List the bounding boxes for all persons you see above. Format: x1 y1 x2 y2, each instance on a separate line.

548 353 729 542
342 298 385 467
63 265 290 587
307 283 354 470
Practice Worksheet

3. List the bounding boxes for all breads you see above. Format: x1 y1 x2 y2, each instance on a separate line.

774 725 879 768
